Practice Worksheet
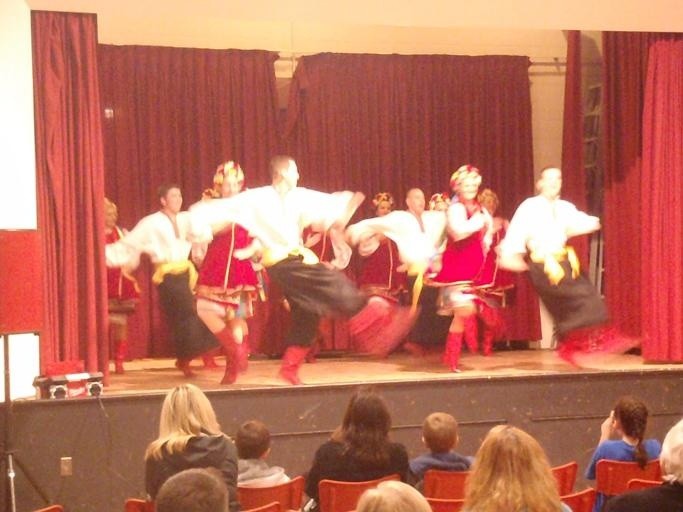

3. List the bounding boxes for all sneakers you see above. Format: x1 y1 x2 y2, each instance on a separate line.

175 359 197 377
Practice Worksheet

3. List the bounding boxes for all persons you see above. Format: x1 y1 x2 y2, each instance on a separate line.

496 165 651 371
346 188 449 365
234 419 291 488
104 182 219 378
303 388 407 511
202 251 268 370
143 383 238 502
194 159 264 386
427 193 450 213
354 480 432 511
422 164 493 372
154 468 229 512
407 411 475 482
599 418 683 512
359 191 430 361
302 226 353 365
103 195 142 375
188 155 419 384
460 424 573 512
584 395 661 511
476 190 515 357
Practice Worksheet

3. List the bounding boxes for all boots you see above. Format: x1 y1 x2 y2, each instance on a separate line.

278 346 309 385
463 314 478 354
113 341 126 374
200 349 214 369
215 326 239 385
478 332 493 356
441 332 463 373
234 335 250 372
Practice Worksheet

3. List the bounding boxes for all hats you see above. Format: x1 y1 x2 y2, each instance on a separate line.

449 164 481 191
212 161 244 185
476 187 498 208
370 192 393 210
429 193 450 210
